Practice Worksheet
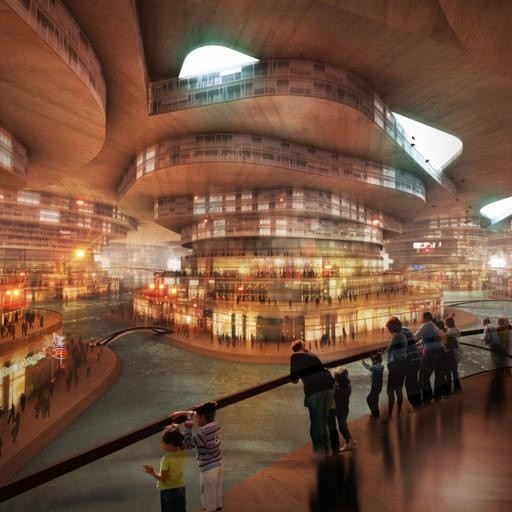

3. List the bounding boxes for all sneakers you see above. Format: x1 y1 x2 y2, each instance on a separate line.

339 438 357 452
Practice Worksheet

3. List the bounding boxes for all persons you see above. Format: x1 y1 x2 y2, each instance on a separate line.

0 247 512 512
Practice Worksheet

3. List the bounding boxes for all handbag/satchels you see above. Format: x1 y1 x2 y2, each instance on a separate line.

453 347 467 365
